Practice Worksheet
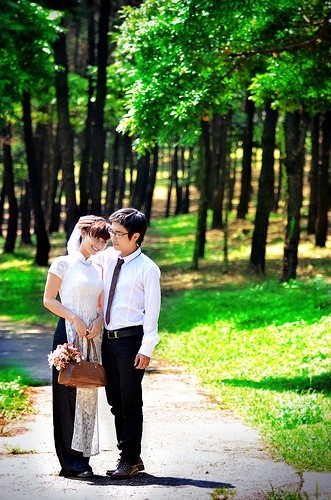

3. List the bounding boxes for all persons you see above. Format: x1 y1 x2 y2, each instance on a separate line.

43 216 110 477
66 208 161 479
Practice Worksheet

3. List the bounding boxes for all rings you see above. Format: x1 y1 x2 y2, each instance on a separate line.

143 365 146 366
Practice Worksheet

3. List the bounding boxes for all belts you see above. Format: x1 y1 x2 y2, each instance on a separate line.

102 326 141 340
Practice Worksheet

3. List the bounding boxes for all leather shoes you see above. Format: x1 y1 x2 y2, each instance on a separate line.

110 459 139 480
106 455 144 475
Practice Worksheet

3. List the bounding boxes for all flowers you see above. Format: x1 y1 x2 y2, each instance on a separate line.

47 342 86 370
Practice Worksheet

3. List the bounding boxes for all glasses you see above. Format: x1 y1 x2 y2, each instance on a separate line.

107 226 129 239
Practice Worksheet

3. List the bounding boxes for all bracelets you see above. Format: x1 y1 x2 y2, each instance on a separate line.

98 314 104 320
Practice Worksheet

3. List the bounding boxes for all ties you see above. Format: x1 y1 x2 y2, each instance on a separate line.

106 257 124 325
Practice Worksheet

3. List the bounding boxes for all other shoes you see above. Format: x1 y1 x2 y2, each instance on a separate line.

77 470 93 478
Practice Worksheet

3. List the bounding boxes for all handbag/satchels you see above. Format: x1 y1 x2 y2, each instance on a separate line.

57 328 109 389
